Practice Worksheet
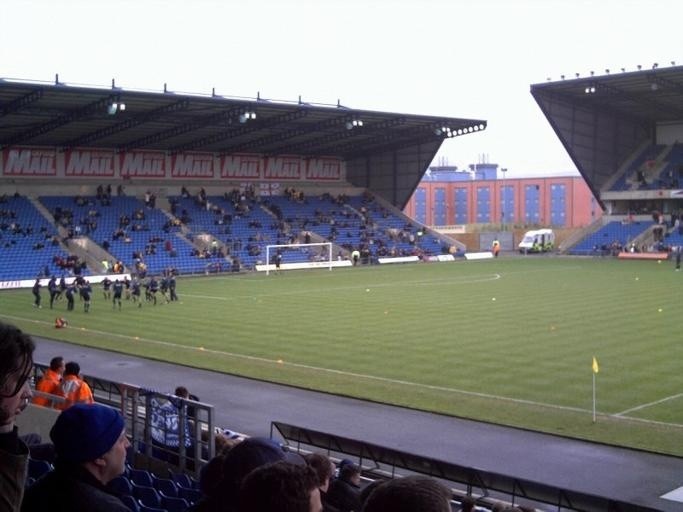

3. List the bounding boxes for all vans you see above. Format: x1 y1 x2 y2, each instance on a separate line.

518 229 554 253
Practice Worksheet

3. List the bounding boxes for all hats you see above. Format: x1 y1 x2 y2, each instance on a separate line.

223 436 307 483
49 404 124 466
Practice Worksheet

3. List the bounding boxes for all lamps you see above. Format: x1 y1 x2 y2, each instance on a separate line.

110 93 126 111
351 112 362 127
441 121 450 132
244 103 257 119
584 83 596 93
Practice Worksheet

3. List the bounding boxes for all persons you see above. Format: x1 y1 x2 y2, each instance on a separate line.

20 403 133 510
1 323 44 512
32 275 177 313
493 243 501 258
188 395 239 443
48 361 96 411
18 432 53 465
0 183 457 275
32 357 66 407
197 434 537 512
152 386 191 449
592 155 683 270
492 238 499 247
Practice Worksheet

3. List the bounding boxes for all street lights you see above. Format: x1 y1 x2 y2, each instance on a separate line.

500 168 507 232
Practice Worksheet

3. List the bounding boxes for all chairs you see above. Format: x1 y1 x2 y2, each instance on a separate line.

568 140 682 253
15 418 257 512
